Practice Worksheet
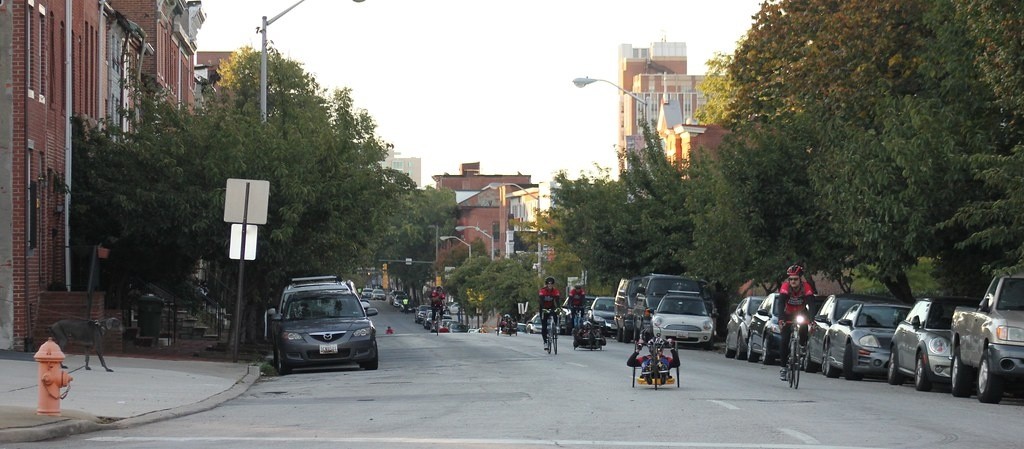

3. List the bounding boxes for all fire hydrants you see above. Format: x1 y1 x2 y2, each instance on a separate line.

35 338 72 415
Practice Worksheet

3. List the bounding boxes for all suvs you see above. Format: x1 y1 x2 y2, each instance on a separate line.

559 297 594 334
265 274 379 372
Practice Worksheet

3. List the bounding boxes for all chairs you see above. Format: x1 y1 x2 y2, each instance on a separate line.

296 303 310 317
334 300 351 316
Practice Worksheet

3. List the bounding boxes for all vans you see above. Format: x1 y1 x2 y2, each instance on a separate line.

613 275 643 342
632 274 700 342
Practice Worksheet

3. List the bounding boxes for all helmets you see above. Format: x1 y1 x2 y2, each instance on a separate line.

575 283 582 289
787 264 804 275
647 337 665 347
436 286 443 290
583 321 592 330
545 277 555 285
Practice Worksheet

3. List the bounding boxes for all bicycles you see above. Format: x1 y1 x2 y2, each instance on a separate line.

435 308 441 336
780 319 810 389
543 308 560 355
572 308 581 332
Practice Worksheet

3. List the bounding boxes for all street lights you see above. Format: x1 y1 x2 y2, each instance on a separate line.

260 0 368 121
489 182 541 277
440 227 494 262
572 75 647 122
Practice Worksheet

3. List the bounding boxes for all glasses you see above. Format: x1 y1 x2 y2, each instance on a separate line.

788 278 800 281
649 345 663 351
547 282 554 284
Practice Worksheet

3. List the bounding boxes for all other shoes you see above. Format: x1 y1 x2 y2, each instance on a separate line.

439 318 443 325
556 326 561 334
544 343 549 351
660 365 667 384
431 323 435 330
779 370 787 380
644 365 652 385
799 344 807 356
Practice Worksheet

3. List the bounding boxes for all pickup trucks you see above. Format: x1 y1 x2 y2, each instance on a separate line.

950 273 1024 403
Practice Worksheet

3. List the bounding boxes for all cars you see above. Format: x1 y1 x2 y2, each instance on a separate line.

650 292 718 350
723 290 785 364
588 296 616 336
361 288 416 312
516 313 565 333
803 292 979 391
415 305 482 333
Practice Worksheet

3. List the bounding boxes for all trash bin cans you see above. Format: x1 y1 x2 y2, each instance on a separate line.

138 296 163 339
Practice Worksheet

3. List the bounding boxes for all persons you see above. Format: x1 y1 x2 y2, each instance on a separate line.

569 284 585 333
357 287 363 299
501 314 517 331
539 276 560 350
402 296 415 315
385 326 395 335
430 285 448 330
779 265 817 381
627 337 681 385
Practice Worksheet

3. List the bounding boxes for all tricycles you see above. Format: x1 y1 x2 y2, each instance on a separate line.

632 333 680 390
573 325 603 351
497 318 518 336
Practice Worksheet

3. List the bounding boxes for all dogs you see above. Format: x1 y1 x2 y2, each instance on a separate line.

47 315 123 372
